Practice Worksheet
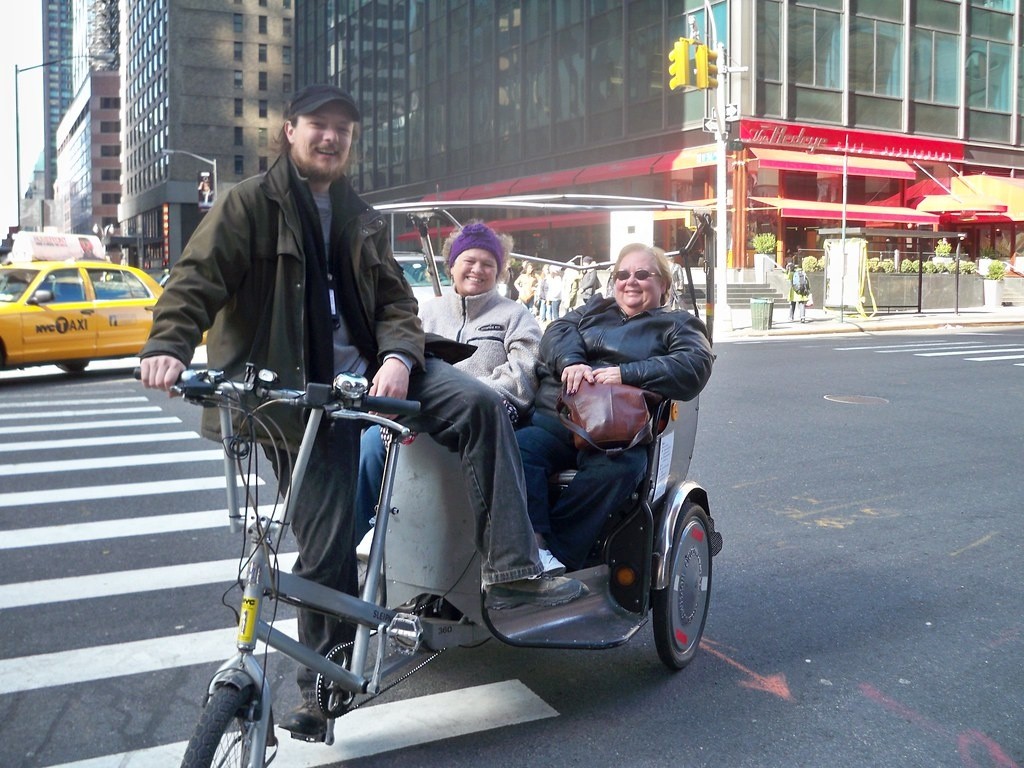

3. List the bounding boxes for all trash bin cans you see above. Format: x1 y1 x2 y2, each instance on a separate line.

749 297 774 332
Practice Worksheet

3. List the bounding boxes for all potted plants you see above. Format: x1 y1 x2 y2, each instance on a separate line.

752 232 778 284
932 237 953 268
983 259 1007 306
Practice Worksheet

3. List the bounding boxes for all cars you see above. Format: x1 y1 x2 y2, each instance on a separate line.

1 232 211 382
390 251 456 310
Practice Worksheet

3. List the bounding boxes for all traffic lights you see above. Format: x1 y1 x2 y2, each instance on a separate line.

668 42 689 92
693 44 719 91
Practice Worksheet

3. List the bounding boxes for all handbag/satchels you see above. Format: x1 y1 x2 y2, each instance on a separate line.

554 373 664 456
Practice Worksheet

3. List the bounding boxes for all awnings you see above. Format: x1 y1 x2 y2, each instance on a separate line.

750 149 939 224
396 146 717 241
951 175 1024 221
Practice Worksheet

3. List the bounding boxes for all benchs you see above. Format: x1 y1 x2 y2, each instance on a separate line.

546 469 581 490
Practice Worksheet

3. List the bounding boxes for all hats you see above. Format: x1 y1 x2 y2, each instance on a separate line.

288 84 361 123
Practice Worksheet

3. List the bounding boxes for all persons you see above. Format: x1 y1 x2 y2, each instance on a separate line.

141 82 587 737
355 218 544 590
787 265 811 322
516 246 714 575
78 238 105 262
506 255 598 322
198 177 212 203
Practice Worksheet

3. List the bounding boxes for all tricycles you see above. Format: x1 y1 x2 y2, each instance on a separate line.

125 188 724 768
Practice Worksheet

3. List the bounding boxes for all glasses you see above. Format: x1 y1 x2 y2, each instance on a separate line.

614 270 660 280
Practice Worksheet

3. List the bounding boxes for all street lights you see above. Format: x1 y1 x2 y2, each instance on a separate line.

92 223 114 253
160 148 217 205
13 50 114 231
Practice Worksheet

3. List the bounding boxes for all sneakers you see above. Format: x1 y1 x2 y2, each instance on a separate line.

279 690 330 734
484 574 589 609
